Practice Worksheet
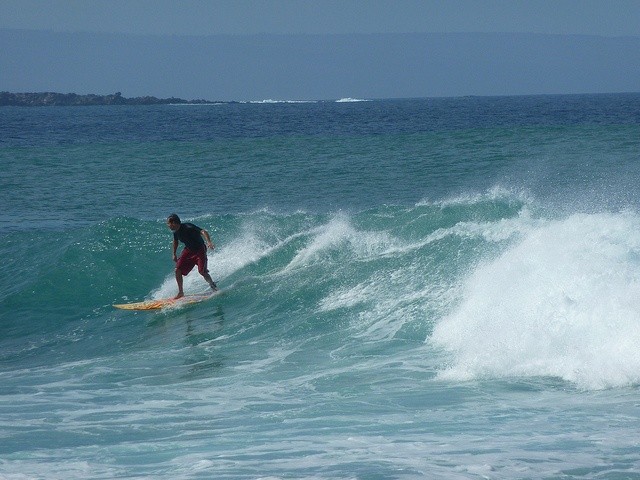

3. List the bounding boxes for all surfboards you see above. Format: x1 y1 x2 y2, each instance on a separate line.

112 295 208 310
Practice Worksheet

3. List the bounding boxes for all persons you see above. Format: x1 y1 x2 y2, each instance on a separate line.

166 213 218 299
